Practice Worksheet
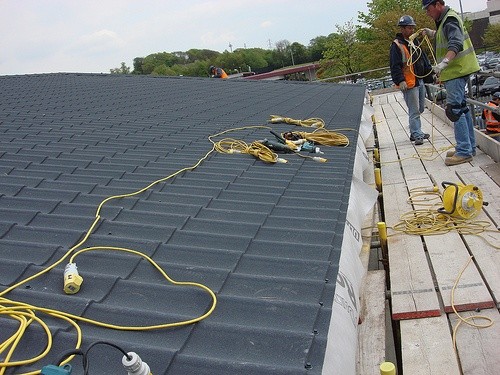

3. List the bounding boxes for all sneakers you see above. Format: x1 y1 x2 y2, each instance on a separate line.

444 151 476 166
410 134 430 145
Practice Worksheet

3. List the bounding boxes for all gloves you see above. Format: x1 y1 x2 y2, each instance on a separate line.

399 81 408 91
422 28 436 37
434 62 448 76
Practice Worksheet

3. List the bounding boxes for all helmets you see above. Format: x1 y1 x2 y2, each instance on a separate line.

493 92 500 98
398 15 417 26
422 0 435 9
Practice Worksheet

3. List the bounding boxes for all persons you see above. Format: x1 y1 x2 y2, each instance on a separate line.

209 66 228 78
389 0 480 166
482 92 500 141
